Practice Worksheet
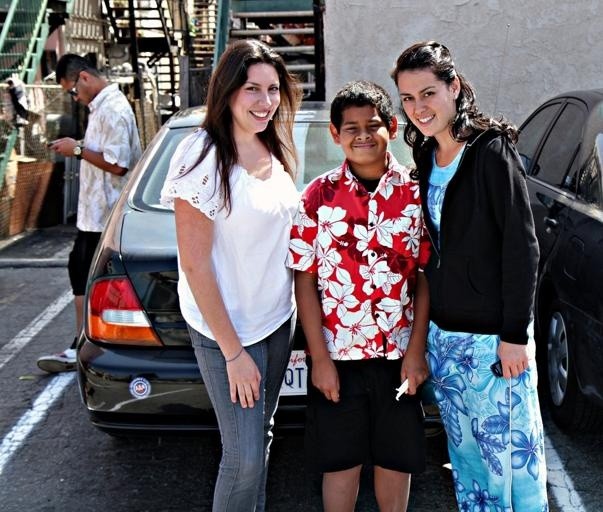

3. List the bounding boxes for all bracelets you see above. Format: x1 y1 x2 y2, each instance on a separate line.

224 345 243 361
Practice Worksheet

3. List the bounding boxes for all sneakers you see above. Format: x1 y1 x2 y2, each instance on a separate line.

37 347 77 373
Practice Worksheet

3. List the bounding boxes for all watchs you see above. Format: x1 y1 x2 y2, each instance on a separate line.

72 145 84 161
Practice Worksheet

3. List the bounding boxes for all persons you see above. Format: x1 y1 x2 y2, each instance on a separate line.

281 81 430 510
37 54 141 371
391 44 551 511
158 39 300 511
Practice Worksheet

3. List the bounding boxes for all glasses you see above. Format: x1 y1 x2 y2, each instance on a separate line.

67 68 87 96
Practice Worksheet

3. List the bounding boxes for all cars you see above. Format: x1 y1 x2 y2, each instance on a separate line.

536 180 603 308
512 87 603 412
76 100 447 442
